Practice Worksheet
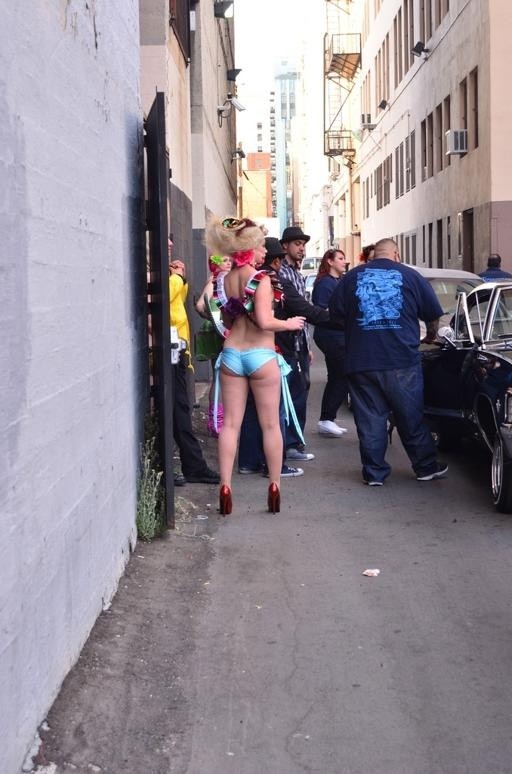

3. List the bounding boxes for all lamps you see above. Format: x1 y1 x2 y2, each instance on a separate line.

379 99 391 110
230 147 246 160
410 41 430 57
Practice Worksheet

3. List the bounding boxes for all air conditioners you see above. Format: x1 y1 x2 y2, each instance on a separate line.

445 128 468 156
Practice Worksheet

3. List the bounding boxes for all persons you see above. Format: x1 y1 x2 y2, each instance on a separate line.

358 243 375 263
311 248 348 437
196 256 232 361
329 237 449 486
145 228 222 487
211 217 308 516
476 253 511 280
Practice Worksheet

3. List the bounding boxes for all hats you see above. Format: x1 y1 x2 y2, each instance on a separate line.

265 237 287 256
279 227 310 243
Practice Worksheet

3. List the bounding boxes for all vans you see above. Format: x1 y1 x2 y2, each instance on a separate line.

301 257 322 279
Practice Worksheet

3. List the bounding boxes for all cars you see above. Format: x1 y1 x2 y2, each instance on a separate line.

407 265 487 338
304 273 319 305
420 280 512 514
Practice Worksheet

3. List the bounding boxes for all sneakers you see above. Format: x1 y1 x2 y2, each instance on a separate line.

240 463 265 474
318 420 342 434
332 422 347 432
417 463 449 480
266 466 303 478
286 448 315 461
173 473 185 486
362 479 384 486
187 470 219 483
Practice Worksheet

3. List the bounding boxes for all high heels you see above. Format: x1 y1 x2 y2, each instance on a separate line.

268 483 280 514
220 485 232 516
